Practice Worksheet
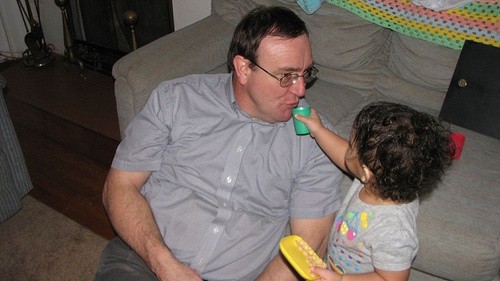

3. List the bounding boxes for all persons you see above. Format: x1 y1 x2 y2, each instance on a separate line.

295 102 456 281
96 7 346 281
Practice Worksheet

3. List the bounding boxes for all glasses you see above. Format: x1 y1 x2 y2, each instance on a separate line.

244 57 318 88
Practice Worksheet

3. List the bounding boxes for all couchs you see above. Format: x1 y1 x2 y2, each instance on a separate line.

112 0 500 281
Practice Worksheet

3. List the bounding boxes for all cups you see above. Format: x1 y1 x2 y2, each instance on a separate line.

447 132 465 159
292 107 311 135
